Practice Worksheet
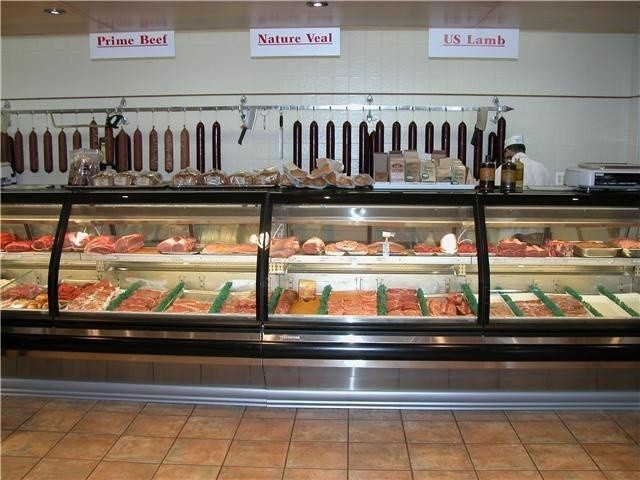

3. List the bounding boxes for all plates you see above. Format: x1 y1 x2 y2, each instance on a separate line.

325 244 497 257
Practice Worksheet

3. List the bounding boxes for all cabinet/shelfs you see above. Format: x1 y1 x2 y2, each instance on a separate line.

0 188 640 329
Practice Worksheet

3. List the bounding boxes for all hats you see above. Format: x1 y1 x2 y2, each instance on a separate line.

504 135 527 150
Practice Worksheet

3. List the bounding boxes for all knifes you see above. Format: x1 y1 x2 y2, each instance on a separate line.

238 107 257 145
471 108 488 145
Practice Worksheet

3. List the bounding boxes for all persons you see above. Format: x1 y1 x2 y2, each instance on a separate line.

494 134 551 240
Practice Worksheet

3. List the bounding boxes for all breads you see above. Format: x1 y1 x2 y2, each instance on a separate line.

69 159 371 187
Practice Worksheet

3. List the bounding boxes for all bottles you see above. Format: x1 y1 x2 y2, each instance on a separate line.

514 156 524 193
479 154 495 194
501 155 516 193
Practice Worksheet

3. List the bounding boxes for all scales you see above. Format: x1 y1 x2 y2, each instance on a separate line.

562 160 640 193
